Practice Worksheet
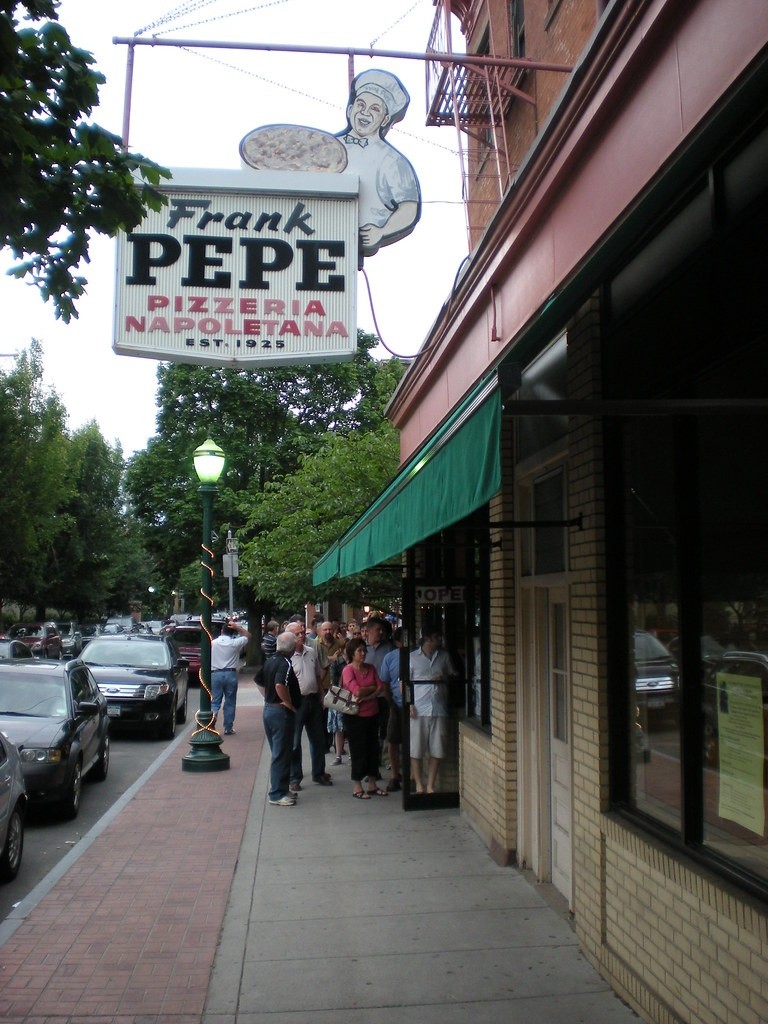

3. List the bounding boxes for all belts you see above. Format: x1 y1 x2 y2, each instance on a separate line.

211 668 236 673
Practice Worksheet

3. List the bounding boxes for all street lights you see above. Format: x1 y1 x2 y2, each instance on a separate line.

181 435 230 772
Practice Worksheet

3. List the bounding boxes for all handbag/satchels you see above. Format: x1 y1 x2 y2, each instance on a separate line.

323 684 360 715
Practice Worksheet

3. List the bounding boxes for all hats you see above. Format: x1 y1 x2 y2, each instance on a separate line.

419 624 438 641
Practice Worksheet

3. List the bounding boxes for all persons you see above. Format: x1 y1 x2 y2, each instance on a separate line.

209 621 252 735
255 611 459 806
635 706 648 755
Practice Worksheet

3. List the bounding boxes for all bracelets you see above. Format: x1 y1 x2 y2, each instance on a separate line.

239 627 242 632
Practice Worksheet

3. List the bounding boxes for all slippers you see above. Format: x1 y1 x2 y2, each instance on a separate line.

367 787 388 796
353 791 371 799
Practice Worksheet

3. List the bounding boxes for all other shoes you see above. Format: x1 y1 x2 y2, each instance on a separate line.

330 758 341 766
285 791 298 798
341 750 346 755
224 729 236 735
386 773 402 791
289 783 302 792
410 779 416 793
312 776 332 786
268 796 295 806
325 773 331 778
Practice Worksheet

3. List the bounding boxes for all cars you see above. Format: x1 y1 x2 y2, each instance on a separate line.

78 633 190 740
106 616 145 634
0 732 27 881
139 610 246 640
0 638 39 659
649 628 677 645
170 625 202 685
81 624 126 647
57 623 83 659
666 633 768 685
0 622 63 660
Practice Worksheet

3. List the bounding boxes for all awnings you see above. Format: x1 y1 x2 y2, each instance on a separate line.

311 365 523 587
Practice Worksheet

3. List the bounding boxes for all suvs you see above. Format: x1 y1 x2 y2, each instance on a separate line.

634 627 678 736
0 656 109 820
704 651 768 789
